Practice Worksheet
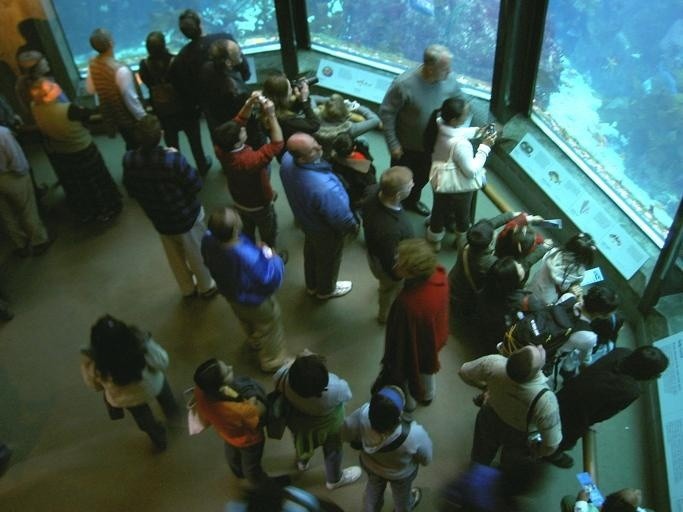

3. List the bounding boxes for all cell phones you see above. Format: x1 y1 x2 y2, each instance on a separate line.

489 124 494 138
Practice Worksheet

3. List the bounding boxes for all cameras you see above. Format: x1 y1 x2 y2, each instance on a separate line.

528 433 542 442
291 77 318 94
254 95 265 103
262 244 273 260
345 103 355 110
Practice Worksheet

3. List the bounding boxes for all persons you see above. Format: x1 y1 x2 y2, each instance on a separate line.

2 8 251 253
81 316 180 451
448 208 670 512
123 43 498 509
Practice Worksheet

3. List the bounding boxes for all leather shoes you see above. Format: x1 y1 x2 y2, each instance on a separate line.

33 232 57 257
13 248 29 257
200 155 211 175
409 201 430 216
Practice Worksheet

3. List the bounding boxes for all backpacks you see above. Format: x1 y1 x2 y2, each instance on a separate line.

142 55 182 118
499 298 597 392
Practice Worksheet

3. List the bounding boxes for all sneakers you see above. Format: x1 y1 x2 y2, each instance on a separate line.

543 451 573 468
326 466 361 489
269 475 291 488
315 281 352 300
410 487 421 507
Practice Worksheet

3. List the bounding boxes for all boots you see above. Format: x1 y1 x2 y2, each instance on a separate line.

453 226 466 250
426 224 445 252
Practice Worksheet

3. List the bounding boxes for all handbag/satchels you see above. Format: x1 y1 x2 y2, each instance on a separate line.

104 390 125 421
429 137 482 194
266 369 290 440
186 397 210 436
